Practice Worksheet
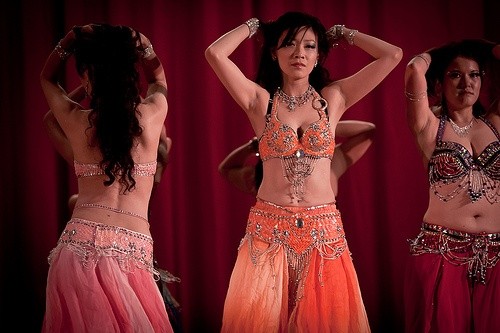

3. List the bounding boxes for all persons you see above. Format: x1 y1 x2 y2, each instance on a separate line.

218 119 376 194
405 39 500 333
41 23 173 333
45 85 184 316
204 10 403 333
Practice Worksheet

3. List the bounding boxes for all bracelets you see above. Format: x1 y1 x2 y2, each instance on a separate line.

56 40 71 60
331 24 358 45
138 43 154 57
417 55 430 67
243 17 259 38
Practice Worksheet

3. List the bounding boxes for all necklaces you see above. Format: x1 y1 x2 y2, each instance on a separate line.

448 117 474 137
280 85 313 110
